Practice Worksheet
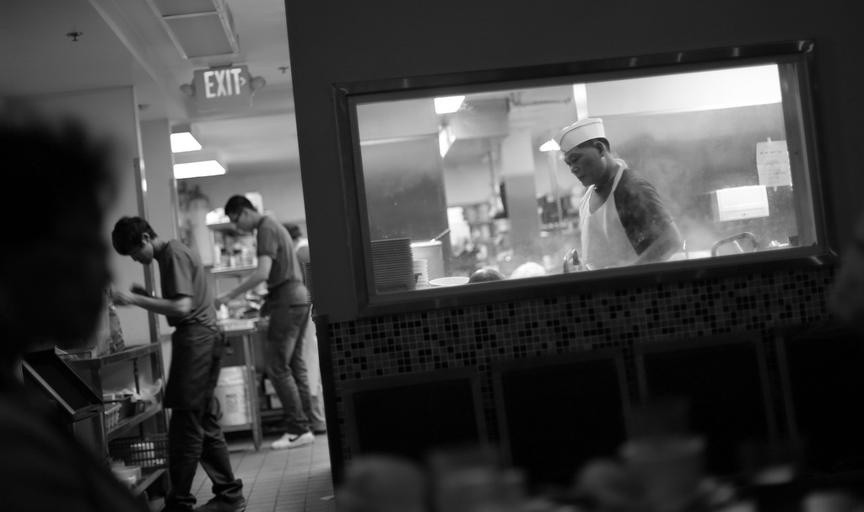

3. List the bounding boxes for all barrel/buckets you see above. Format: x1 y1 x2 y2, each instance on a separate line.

211 365 256 425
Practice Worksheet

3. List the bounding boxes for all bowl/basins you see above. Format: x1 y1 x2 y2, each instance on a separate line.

110 439 158 487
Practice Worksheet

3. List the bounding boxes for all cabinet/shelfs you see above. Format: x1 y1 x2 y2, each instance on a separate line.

66 340 170 506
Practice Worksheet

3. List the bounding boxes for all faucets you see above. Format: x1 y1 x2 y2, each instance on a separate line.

562 249 581 273
709 232 759 257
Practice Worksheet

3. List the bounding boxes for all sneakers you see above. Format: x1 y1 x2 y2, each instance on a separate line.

195 496 245 512
271 431 314 449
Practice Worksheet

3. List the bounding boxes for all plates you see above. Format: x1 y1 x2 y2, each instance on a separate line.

431 274 471 288
371 237 430 293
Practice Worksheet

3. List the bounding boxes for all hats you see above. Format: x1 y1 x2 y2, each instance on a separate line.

554 118 606 152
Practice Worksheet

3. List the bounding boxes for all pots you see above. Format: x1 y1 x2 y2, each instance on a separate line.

410 240 444 282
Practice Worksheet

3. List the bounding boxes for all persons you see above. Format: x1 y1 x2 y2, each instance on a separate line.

552 117 684 268
213 194 315 452
0 91 153 510
218 233 241 255
282 221 327 435
111 213 247 511
